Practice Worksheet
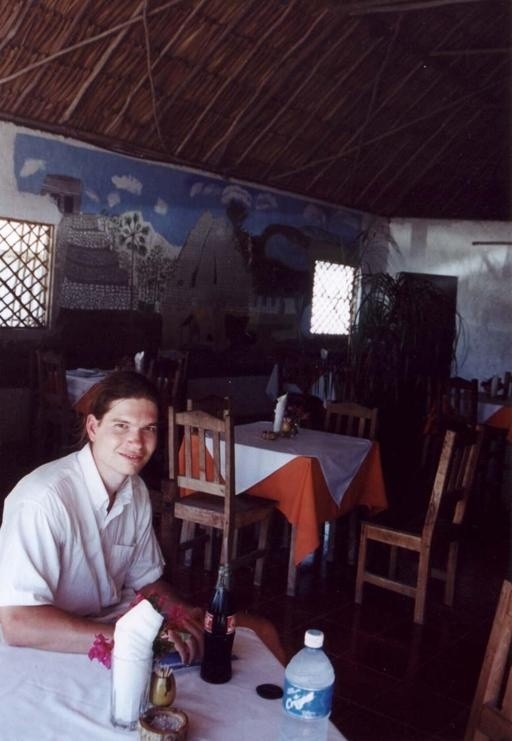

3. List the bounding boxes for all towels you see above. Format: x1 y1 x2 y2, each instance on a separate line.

112 598 165 723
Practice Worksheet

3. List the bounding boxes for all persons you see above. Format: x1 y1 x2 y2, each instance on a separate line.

1 371 208 667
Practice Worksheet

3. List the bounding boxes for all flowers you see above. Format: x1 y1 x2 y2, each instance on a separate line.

88 633 172 668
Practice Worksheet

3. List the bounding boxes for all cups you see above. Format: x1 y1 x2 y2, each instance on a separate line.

109 649 152 728
273 411 283 434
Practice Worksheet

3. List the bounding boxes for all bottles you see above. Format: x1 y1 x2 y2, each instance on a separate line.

149 672 176 707
275 630 337 741
199 566 235 683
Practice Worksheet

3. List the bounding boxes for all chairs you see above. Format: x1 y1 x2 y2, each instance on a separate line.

462 579 512 741
30 349 512 625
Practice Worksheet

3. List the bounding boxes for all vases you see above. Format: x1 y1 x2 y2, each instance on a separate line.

150 660 160 702
